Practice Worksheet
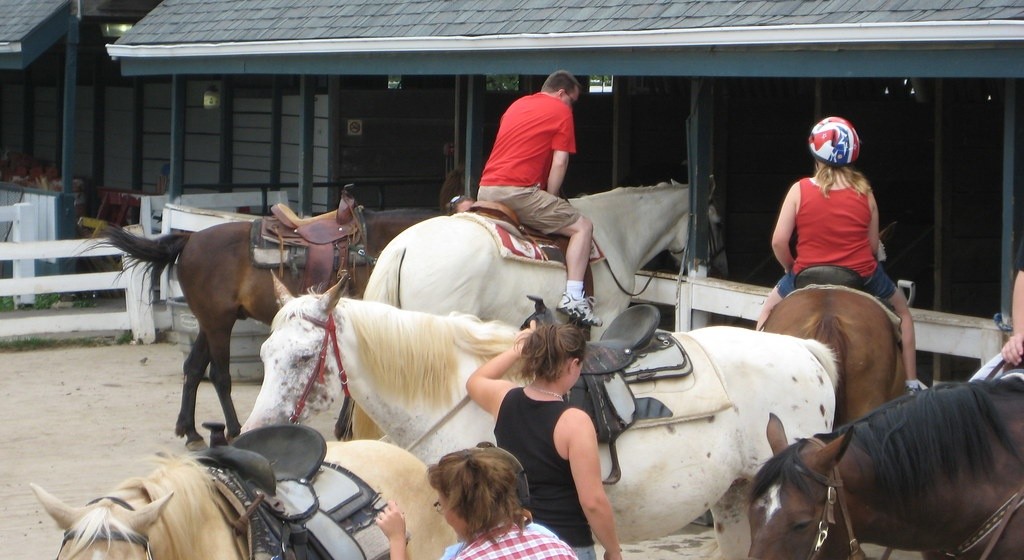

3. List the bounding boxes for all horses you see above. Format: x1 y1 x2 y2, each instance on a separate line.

28 168 1024 559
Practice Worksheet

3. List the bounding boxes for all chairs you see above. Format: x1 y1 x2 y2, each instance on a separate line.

154 164 182 195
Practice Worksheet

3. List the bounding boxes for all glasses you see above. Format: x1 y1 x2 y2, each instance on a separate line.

433 500 455 516
449 195 461 211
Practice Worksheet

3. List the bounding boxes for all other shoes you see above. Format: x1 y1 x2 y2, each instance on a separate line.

905 382 928 395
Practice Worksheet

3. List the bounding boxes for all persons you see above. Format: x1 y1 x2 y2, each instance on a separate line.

755 116 927 397
466 320 623 560
477 69 602 326
448 196 475 214
375 444 579 560
963 241 1024 384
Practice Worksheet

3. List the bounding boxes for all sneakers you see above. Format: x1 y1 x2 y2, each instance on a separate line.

556 291 603 327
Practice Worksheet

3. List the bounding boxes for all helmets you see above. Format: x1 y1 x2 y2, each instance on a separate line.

808 116 860 167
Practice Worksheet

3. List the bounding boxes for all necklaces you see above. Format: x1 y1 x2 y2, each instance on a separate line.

530 385 564 402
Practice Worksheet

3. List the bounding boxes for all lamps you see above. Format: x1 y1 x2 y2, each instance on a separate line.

203 80 219 110
